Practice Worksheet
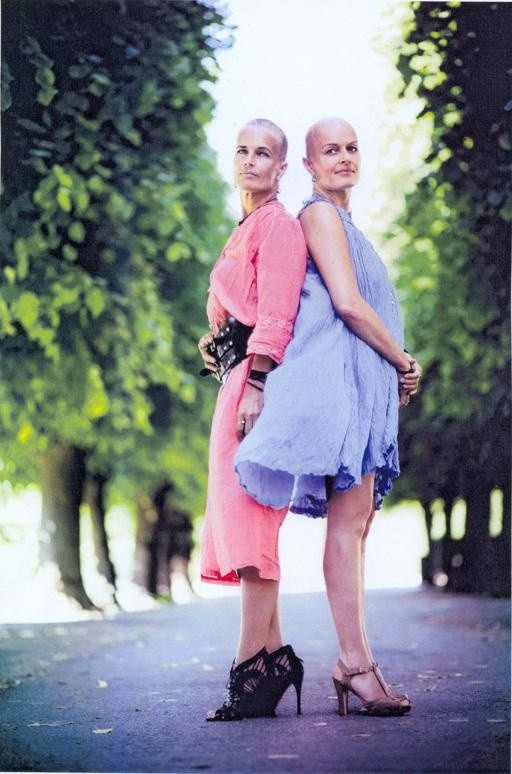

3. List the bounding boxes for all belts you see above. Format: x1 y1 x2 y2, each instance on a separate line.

199 316 254 383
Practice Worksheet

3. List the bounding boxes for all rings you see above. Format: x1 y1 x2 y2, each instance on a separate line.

237 419 245 423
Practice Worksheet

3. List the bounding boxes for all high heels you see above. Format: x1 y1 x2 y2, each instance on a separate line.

206 644 304 720
332 658 411 716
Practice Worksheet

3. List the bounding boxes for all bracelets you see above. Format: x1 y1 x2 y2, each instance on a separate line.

248 368 268 384
246 381 264 392
396 367 414 373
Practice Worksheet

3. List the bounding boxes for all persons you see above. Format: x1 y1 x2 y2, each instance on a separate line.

196 116 308 721
234 115 422 714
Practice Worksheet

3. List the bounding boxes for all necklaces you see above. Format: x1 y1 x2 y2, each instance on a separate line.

238 196 277 225
